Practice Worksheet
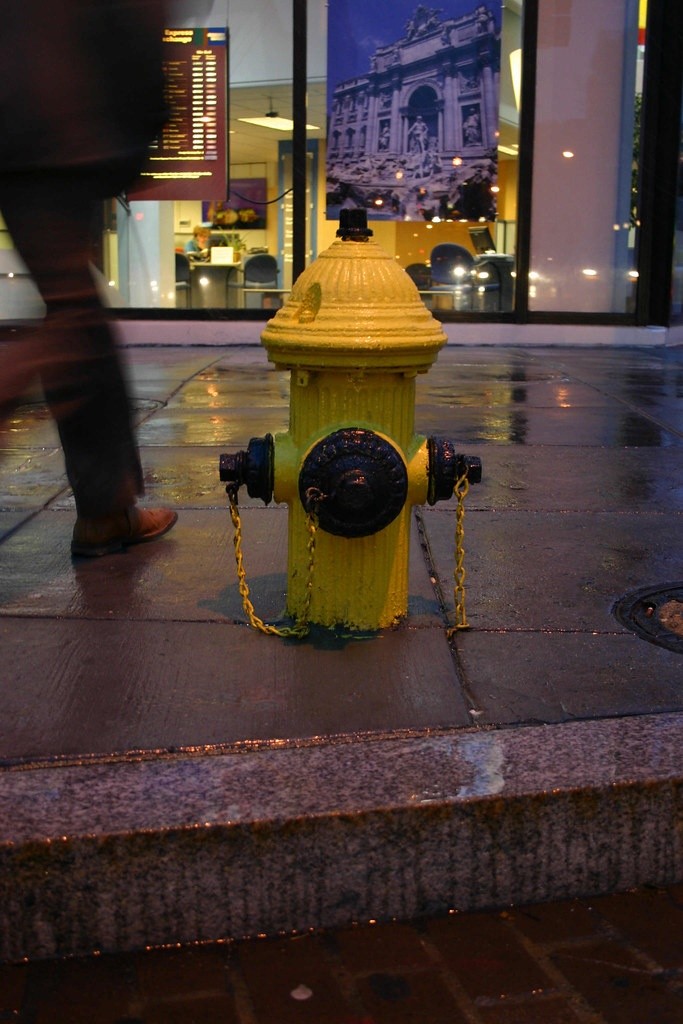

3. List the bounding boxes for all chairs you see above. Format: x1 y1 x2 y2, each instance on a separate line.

401 245 504 314
175 253 279 309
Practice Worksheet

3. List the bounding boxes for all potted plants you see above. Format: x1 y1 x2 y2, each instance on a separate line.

221 229 250 263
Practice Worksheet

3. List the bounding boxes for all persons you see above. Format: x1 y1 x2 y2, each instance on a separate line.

410 153 443 181
464 108 480 143
408 115 429 156
185 225 210 285
405 5 440 40
0 1 179 559
380 122 390 147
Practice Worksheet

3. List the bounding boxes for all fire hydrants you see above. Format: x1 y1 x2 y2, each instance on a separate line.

218 206 485 640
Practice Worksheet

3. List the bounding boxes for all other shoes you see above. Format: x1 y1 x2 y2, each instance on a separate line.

70 503 178 558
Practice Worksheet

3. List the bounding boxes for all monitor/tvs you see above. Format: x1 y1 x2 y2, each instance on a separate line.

469 226 496 255
207 233 238 255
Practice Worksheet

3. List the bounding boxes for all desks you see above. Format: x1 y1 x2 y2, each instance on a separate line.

189 261 241 308
